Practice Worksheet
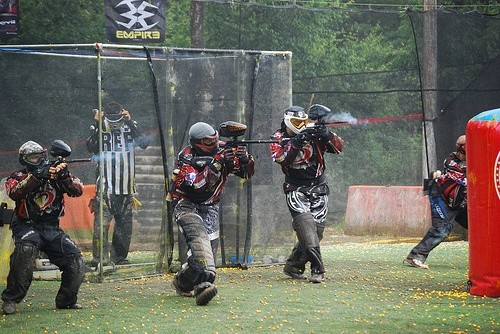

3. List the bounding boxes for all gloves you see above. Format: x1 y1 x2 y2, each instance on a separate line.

284 106 308 135
236 146 249 164
209 148 235 174
290 132 307 149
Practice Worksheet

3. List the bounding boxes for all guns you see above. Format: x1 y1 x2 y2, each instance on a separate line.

42 138 92 169
192 121 298 176
281 103 350 145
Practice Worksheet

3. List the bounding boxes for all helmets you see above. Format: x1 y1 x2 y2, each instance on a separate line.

104 101 124 129
189 122 219 152
18 141 44 166
456 135 465 155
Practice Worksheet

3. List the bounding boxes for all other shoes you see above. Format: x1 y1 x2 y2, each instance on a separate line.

2 299 16 314
65 303 82 309
196 286 218 306
90 257 110 266
312 273 324 282
405 257 428 269
284 269 307 279
116 260 131 265
172 275 194 297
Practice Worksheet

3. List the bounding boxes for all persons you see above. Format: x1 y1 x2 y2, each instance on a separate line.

0 141 84 314
167 121 254 305
270 106 344 283
86 102 149 268
406 135 468 266
